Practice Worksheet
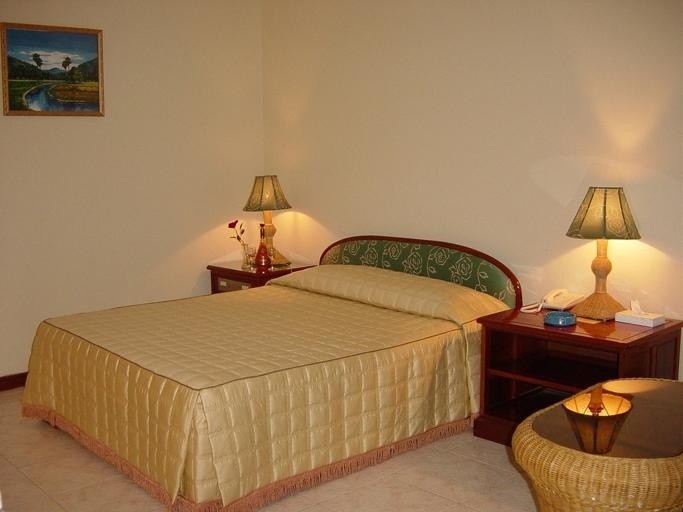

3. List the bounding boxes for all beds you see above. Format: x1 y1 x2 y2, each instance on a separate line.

19 235 522 512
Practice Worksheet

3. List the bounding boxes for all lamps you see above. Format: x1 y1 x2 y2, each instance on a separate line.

242 175 290 265
564 187 642 324
562 385 633 453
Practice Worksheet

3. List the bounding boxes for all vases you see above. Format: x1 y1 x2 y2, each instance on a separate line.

241 244 251 269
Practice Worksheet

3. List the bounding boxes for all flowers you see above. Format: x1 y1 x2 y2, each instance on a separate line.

227 220 248 264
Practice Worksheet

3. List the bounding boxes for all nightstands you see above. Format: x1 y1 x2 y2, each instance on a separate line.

473 302 682 447
206 261 317 295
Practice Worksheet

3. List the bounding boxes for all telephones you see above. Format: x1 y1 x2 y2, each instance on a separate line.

538 288 584 311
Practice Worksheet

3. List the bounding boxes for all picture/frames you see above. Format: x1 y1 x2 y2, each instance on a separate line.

0 22 104 118
